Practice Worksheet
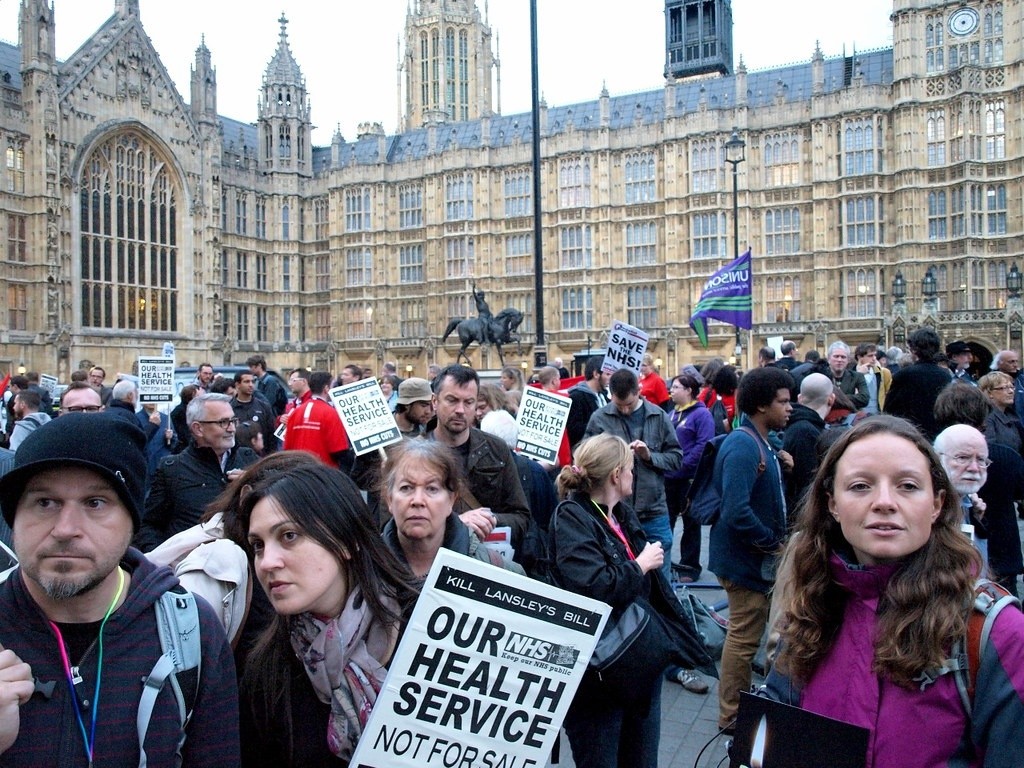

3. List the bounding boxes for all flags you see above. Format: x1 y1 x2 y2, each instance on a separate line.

687 249 752 348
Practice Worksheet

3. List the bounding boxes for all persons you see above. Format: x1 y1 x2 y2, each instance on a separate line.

0 327 912 582
709 367 795 736
932 423 993 549
979 372 1024 455
470 278 494 345
946 339 976 383
990 350 1024 425
1 410 243 767
234 463 429 768
884 328 951 440
750 410 1024 768
931 378 1023 601
537 433 666 768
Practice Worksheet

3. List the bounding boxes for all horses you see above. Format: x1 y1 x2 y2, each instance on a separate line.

442 308 524 369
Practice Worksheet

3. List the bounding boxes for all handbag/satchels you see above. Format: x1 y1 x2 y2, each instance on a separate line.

591 595 673 706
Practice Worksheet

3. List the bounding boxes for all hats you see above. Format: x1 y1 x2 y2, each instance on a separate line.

945 339 970 356
0 405 149 541
395 376 436 406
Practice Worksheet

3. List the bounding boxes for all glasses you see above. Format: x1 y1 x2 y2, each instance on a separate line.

198 416 240 431
91 374 103 379
670 384 686 391
287 377 305 384
58 405 101 415
942 451 993 470
993 385 1016 393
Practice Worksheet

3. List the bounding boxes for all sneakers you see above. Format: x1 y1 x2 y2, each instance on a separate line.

676 669 709 697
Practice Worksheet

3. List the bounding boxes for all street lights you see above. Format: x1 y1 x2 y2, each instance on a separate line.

723 123 745 377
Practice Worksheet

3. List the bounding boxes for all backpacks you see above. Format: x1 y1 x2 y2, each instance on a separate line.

274 382 288 416
673 425 767 532
676 582 733 664
706 389 729 439
